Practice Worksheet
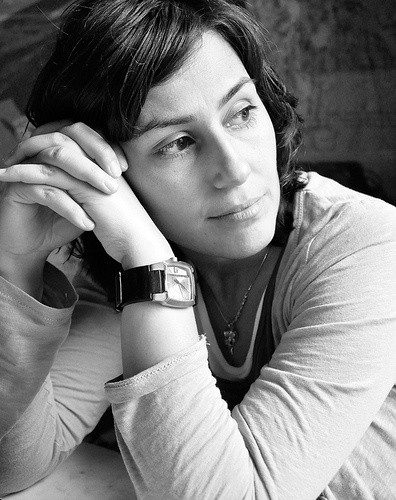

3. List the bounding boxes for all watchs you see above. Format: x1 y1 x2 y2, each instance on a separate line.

114 257 198 313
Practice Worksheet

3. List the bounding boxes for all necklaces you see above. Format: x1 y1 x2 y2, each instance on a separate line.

196 244 272 356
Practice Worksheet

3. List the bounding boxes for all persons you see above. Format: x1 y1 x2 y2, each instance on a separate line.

1 1 396 500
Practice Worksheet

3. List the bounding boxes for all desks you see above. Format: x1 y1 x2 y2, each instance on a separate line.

1 434 324 500
